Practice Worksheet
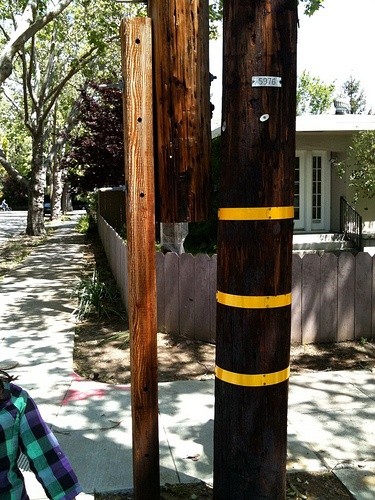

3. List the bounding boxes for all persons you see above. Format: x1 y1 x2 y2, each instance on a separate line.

2 199 8 210
0 370 83 500
